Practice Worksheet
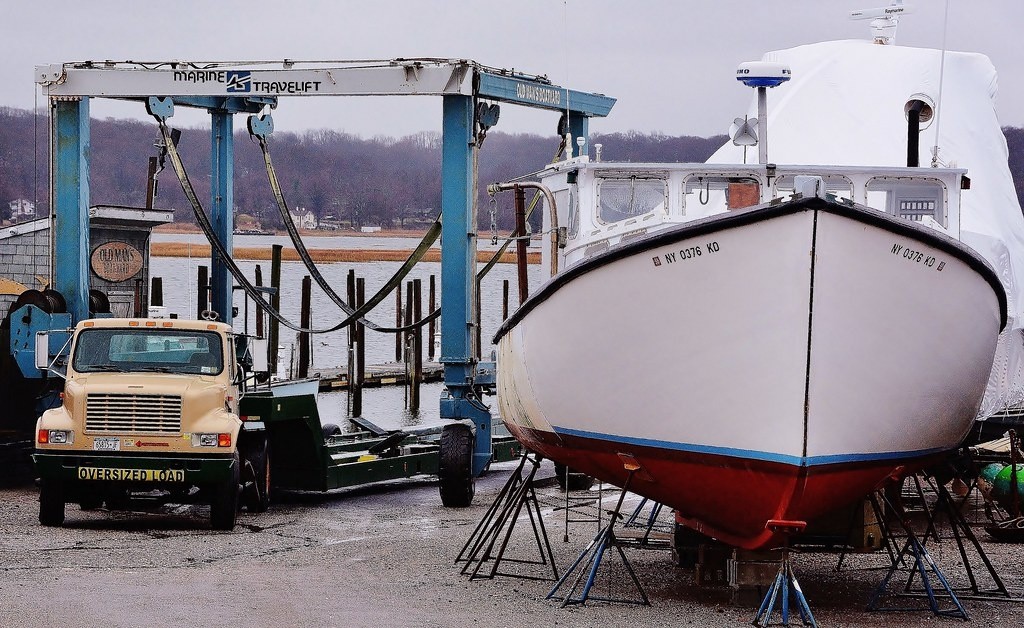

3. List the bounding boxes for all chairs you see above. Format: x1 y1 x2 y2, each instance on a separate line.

189 352 215 368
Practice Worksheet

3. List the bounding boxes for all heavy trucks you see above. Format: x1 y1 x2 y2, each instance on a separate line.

31 61 617 529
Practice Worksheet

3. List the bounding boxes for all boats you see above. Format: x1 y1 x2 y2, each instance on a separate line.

456 0 1024 553
